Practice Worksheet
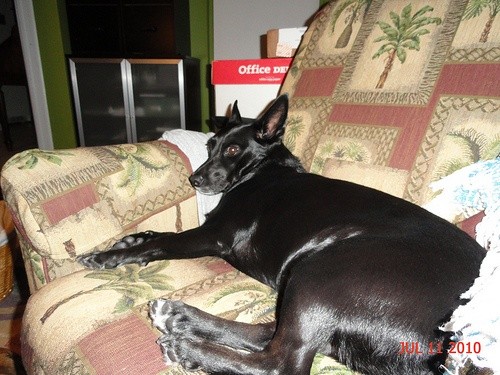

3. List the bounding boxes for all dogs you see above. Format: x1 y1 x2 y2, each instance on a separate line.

74 93 488 375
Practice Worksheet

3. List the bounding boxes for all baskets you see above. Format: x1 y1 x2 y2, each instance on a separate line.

0 201 19 299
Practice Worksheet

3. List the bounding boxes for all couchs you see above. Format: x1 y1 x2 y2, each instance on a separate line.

0 0 500 375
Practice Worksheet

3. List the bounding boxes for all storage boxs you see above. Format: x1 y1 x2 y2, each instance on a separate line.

212 26 308 121
